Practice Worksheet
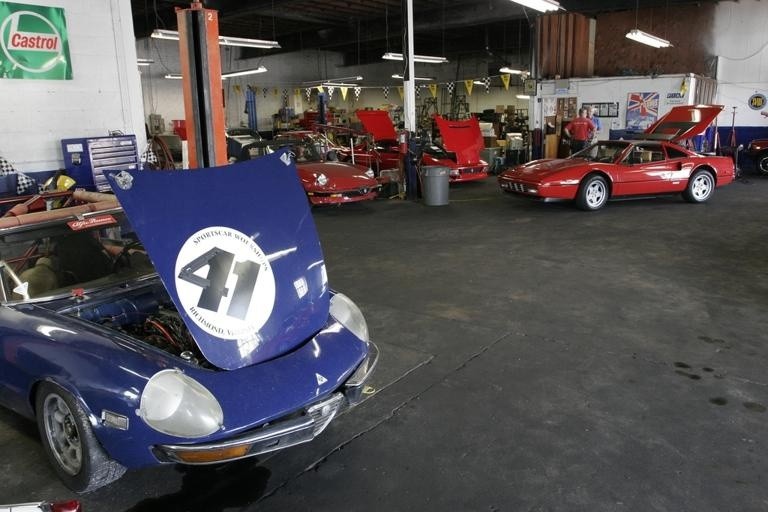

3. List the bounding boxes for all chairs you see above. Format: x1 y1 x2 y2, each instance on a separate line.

14 266 60 298
598 155 643 164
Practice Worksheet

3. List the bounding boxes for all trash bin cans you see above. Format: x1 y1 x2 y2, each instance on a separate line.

420 165 450 207
380 168 399 198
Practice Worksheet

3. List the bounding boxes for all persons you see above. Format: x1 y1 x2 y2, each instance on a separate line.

562 107 595 160
10 237 105 299
586 105 604 159
402 131 420 202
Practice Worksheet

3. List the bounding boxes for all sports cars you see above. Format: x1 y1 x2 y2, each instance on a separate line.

336 110 490 185
748 111 768 174
225 127 267 158
271 129 383 206
496 103 736 213
0 142 383 497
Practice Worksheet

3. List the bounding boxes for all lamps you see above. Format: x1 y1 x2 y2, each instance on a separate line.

498 1 675 76
301 1 450 89
151 0 281 83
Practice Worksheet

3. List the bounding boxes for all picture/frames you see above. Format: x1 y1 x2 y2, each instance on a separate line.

581 102 619 118
